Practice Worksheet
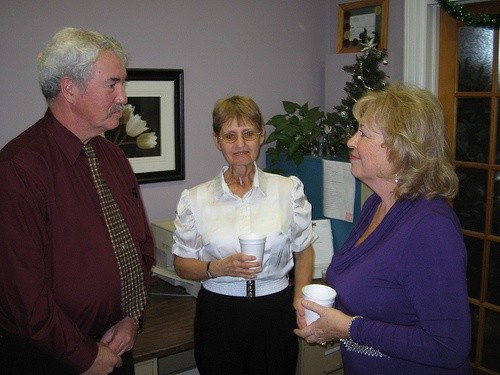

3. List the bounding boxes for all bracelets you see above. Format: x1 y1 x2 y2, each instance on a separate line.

206 260 217 279
347 315 364 346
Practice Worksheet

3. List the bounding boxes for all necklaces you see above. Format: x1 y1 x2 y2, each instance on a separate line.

238 176 245 185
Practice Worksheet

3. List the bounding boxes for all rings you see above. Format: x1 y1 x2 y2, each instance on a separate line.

321 341 327 346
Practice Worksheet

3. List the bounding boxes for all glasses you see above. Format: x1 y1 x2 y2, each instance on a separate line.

217 128 263 144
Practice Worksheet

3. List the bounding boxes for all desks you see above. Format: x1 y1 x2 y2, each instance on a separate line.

130 271 197 375
265 147 375 254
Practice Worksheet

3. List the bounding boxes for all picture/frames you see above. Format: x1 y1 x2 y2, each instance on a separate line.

98 68 185 185
336 0 389 54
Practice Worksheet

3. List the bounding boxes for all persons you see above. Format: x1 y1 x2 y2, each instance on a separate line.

0 27 157 375
293 80 472 375
170 95 318 375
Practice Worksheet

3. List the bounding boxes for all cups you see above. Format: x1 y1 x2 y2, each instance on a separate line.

238 232 267 271
301 284 337 326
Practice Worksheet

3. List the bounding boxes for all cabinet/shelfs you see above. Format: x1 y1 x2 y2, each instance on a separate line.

288 264 344 375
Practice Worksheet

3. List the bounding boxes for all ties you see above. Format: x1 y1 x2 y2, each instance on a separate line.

83 145 148 321
